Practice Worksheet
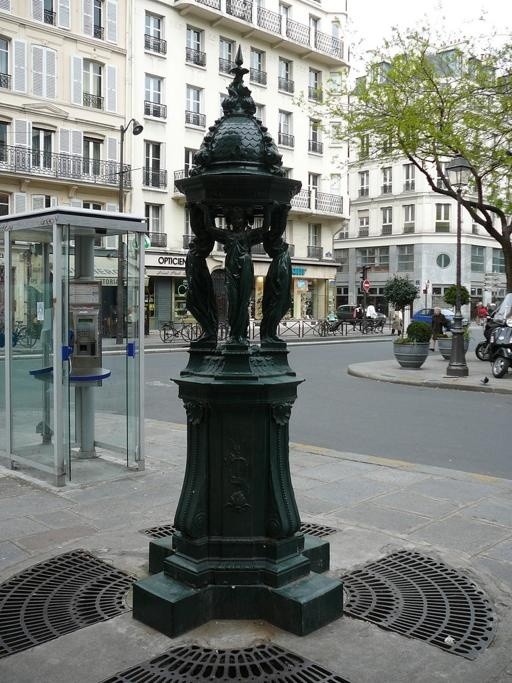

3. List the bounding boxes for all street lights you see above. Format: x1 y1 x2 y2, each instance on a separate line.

445 154 474 378
115 119 143 344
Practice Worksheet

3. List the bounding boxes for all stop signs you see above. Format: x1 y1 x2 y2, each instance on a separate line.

363 280 370 290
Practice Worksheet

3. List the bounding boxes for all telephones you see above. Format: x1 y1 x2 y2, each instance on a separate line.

68 309 98 358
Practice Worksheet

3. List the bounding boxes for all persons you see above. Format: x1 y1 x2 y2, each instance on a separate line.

429 307 448 352
352 306 357 331
391 313 402 336
358 308 362 319
375 302 384 314
201 204 265 345
475 297 501 326
329 310 337 321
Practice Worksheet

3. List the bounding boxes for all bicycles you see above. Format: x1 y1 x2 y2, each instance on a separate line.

159 315 195 343
12 321 38 348
188 319 229 340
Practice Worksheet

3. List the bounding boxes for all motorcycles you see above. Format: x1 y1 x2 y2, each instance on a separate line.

475 292 512 378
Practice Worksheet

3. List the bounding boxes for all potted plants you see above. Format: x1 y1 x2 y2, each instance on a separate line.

392 319 471 369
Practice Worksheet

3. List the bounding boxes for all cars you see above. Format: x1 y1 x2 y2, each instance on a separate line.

412 307 470 331
336 304 387 326
